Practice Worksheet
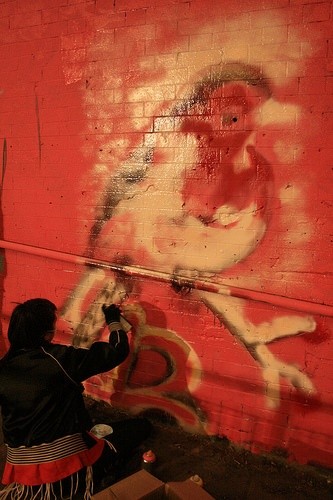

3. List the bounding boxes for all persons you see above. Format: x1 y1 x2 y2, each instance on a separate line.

0 299 152 499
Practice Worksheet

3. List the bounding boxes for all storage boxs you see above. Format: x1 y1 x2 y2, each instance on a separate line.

90 469 215 499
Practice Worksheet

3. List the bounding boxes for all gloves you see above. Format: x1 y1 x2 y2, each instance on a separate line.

101 302 121 325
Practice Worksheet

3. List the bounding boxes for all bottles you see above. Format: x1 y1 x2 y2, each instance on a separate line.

106 305 133 333
190 475 203 487
142 450 156 473
